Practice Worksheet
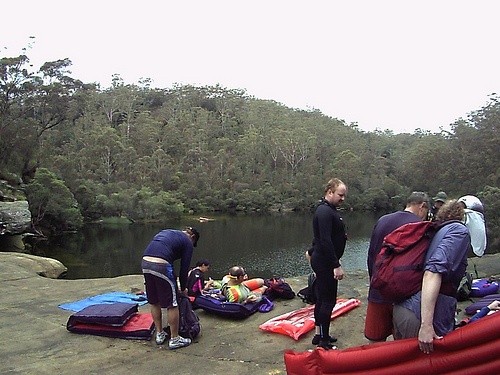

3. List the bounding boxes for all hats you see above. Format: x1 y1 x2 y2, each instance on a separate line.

432 192 449 204
187 227 200 247
458 195 485 214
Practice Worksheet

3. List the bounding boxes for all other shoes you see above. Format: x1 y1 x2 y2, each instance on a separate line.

312 334 336 344
317 340 339 351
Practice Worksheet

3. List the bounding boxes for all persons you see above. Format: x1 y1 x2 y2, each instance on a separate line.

187 259 213 298
364 191 487 354
221 265 268 304
141 225 200 349
306 178 348 350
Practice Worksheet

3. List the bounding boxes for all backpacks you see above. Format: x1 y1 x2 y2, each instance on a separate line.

369 220 470 303
177 290 201 341
265 276 295 300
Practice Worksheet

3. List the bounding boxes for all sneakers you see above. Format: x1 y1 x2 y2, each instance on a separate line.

156 330 168 345
168 335 191 350
